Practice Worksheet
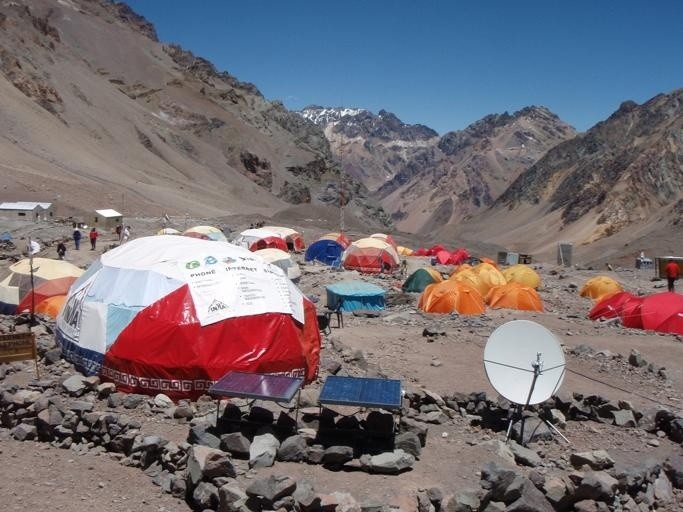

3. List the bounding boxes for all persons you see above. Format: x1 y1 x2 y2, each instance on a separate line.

57 241 66 260
117 224 123 240
90 227 97 250
74 229 81 250
666 260 680 292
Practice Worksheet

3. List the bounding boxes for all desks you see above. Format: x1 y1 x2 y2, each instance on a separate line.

206 371 301 435
314 375 406 435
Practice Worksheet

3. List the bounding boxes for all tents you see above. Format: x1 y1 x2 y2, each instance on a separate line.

54 236 321 402
580 276 683 334
0 258 84 313
15 277 73 320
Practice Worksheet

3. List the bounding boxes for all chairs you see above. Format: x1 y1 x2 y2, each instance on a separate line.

325 298 344 328
